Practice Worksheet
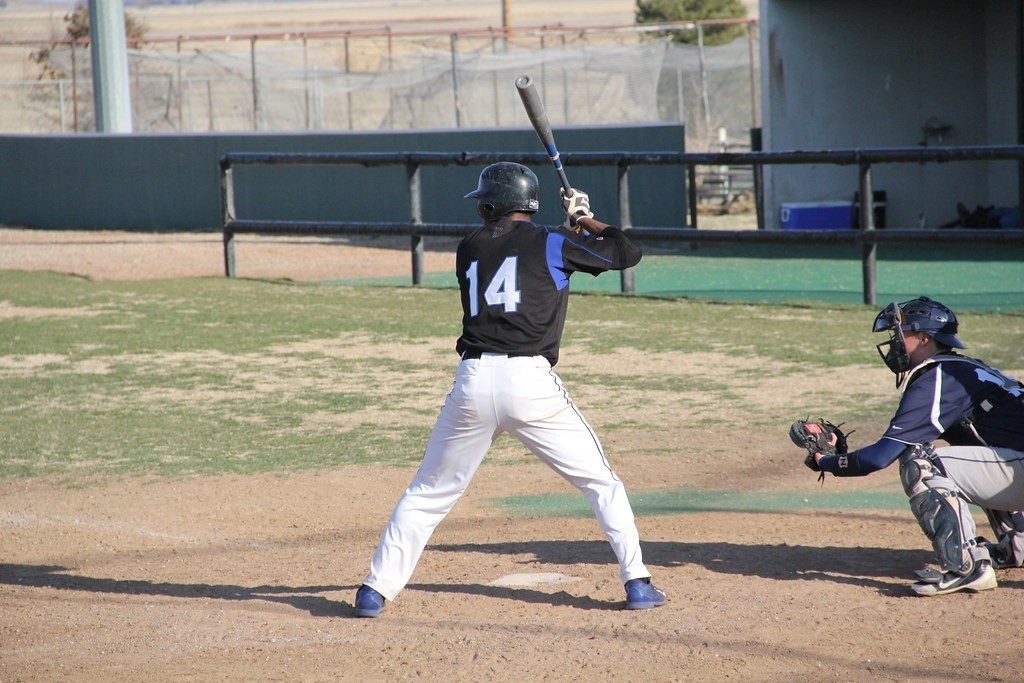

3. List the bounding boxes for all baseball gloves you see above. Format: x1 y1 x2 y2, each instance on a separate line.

789 413 858 488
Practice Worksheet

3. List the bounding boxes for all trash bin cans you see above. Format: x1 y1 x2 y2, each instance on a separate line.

853 190 886 228
780 200 853 229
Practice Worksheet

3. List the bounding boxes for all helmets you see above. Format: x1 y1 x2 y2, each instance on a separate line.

900 298 967 349
463 161 540 222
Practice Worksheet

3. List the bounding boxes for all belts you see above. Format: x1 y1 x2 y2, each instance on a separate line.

462 347 534 362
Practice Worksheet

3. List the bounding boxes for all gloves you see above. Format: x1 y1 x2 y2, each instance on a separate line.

563 216 580 232
560 186 594 225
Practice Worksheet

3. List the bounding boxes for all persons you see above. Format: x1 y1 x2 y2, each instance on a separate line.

356 163 667 618
789 295 1024 594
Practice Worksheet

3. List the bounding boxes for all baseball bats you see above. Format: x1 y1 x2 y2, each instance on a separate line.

514 71 575 199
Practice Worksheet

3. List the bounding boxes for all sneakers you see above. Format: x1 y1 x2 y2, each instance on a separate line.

354 585 386 616
626 579 667 609
908 559 997 597
990 549 1024 569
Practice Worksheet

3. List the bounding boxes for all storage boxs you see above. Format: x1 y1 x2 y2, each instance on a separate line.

780 200 853 230
